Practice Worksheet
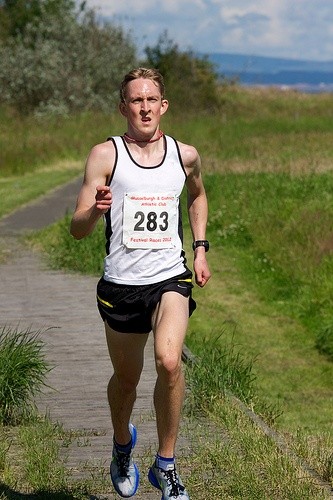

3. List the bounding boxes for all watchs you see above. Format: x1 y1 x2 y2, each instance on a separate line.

192 240 210 252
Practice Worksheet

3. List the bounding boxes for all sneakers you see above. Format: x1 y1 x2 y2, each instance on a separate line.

148 455 190 500
110 423 140 497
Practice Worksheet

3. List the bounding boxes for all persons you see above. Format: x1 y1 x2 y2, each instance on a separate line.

70 65 212 499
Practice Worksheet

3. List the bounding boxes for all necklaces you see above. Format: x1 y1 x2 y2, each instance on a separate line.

124 131 164 142
